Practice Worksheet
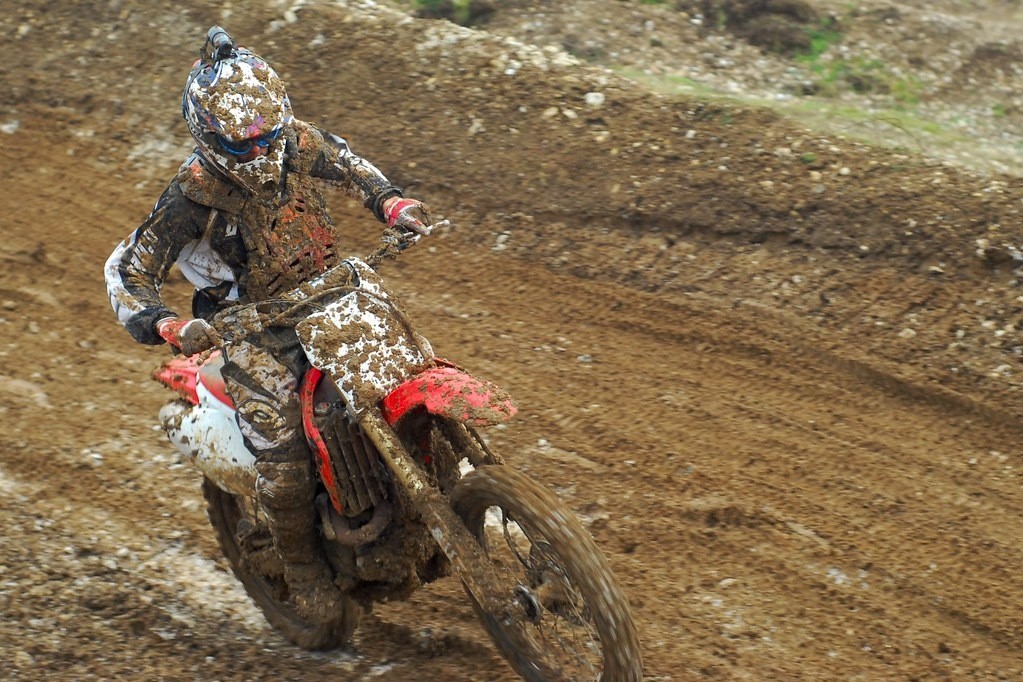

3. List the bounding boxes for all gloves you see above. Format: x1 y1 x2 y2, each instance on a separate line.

155 316 223 357
384 198 433 236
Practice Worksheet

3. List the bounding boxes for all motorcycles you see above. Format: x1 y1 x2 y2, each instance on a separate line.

150 220 644 682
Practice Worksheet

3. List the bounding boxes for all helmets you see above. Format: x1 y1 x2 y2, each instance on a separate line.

182 46 293 197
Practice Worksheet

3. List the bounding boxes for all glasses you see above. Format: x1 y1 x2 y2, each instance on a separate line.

217 128 279 154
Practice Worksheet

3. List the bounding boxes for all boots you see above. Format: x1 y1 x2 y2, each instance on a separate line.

260 502 342 624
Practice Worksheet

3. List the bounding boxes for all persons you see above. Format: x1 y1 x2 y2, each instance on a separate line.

103 27 437 625
